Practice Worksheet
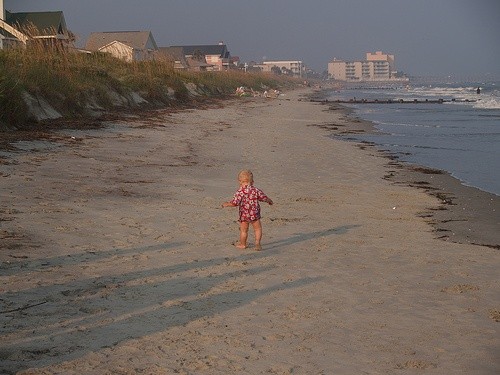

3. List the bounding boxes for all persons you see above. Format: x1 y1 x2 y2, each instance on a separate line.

263 80 311 98
223 168 274 250
